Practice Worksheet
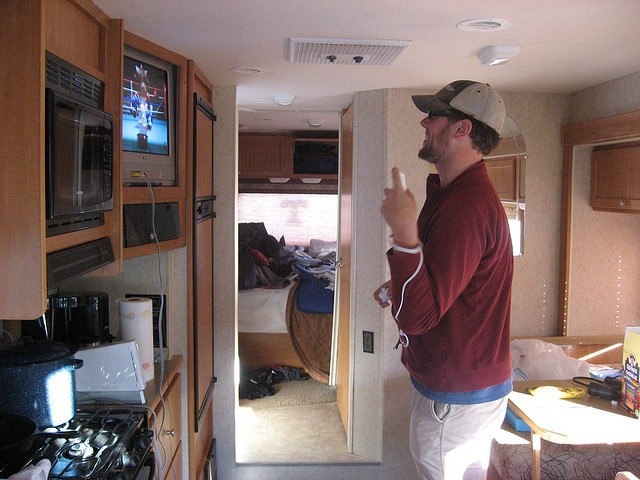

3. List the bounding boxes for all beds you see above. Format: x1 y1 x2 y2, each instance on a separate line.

239 244 336 381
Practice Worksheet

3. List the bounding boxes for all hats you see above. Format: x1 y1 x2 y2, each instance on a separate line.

412 80 505 135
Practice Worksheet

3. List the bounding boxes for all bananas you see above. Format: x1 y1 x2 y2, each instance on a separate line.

526 386 587 399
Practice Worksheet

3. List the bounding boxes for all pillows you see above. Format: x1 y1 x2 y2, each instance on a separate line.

308 238 336 256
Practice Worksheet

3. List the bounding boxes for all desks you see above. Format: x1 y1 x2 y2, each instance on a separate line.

505 378 640 480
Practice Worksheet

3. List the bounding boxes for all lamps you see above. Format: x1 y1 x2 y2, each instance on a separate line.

475 44 518 68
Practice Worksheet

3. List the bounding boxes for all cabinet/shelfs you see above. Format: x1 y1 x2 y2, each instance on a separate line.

236 130 340 194
0 0 124 321
122 27 185 261
141 354 184 480
591 140 640 212
481 134 517 206
186 58 218 480
515 134 526 203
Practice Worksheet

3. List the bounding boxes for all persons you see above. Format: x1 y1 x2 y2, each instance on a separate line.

371 77 516 480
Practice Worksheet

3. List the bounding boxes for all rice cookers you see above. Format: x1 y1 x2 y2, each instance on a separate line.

0 334 85 432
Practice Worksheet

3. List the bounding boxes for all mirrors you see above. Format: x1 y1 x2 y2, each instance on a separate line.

429 106 526 256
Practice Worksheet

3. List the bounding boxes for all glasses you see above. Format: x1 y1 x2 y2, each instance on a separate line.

427 110 461 119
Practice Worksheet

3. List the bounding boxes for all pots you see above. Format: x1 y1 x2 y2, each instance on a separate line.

0 413 79 467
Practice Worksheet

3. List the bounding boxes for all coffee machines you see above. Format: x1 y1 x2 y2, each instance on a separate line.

23 289 116 347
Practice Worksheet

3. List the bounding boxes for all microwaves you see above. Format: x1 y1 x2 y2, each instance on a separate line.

46 90 116 220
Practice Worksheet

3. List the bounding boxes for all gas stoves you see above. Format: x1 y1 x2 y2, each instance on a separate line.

1 409 158 480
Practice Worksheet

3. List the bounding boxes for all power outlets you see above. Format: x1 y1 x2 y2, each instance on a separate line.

363 330 374 354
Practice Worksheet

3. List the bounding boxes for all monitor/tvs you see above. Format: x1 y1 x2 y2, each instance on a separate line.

121 43 178 187
